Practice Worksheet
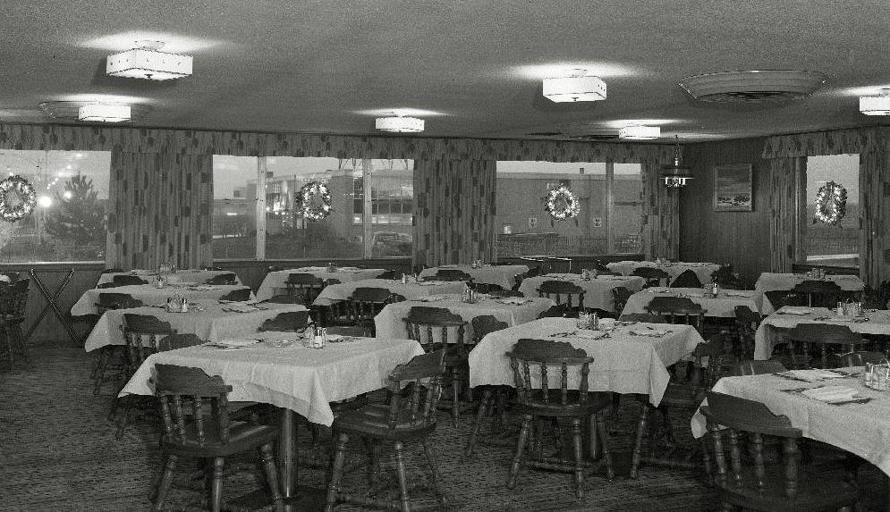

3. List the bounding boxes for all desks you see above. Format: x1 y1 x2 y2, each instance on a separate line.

2 261 105 347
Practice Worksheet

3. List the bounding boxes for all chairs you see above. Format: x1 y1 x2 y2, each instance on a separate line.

0 279 33 372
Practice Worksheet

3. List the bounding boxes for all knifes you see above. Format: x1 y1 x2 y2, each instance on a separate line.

781 384 825 392
772 371 811 383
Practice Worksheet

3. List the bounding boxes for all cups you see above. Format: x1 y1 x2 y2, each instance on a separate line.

472 259 476 269
476 259 482 268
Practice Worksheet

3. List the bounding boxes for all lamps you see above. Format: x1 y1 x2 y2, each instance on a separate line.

619 123 660 140
658 136 699 192
78 101 131 124
859 89 889 118
105 41 194 82
543 70 607 102
375 114 425 134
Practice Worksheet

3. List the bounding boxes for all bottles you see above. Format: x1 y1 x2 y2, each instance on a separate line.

806 268 825 279
152 274 163 289
865 362 888 392
167 293 188 312
462 289 469 303
577 311 598 330
712 283 718 297
303 321 327 349
837 301 863 318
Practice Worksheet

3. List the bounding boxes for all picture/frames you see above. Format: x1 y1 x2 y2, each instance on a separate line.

712 163 753 212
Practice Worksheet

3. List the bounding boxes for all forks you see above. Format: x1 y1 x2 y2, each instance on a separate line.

828 398 871 406
